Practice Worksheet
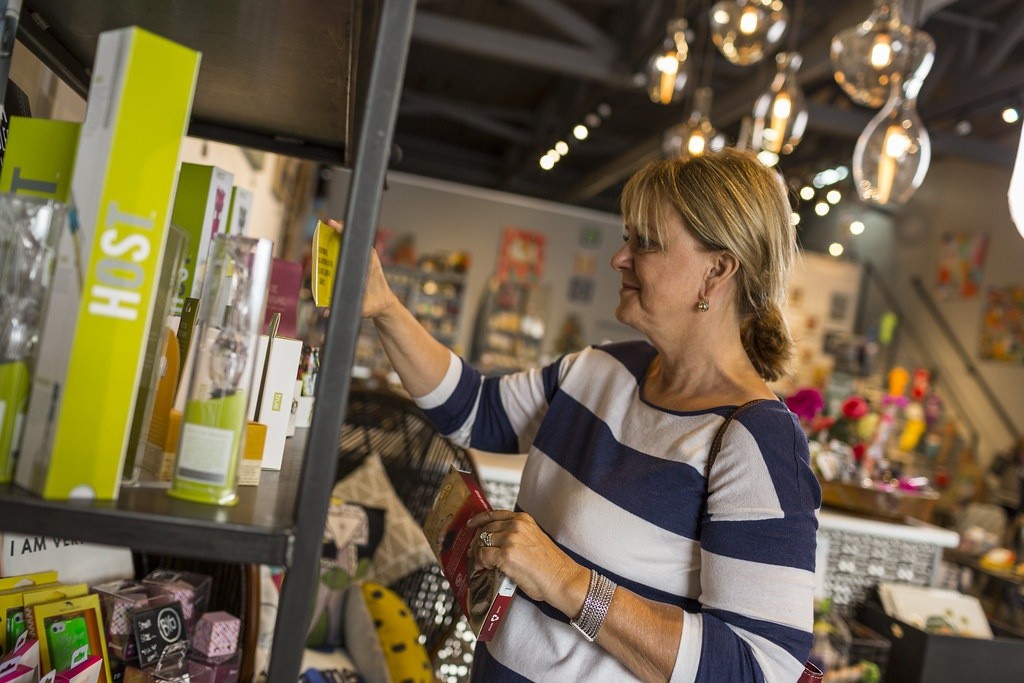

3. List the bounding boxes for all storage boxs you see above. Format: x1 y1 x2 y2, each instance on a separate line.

0 24 255 498
855 599 1024 683
423 463 516 644
472 281 551 377
91 568 243 683
353 263 465 368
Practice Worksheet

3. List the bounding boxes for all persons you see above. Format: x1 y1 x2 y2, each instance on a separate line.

327 145 822 683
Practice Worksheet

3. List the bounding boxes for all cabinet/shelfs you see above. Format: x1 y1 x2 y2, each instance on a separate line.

0 0 416 683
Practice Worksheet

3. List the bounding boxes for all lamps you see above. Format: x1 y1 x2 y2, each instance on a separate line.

646 0 935 204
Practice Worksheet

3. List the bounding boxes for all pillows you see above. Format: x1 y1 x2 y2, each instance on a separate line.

251 453 438 683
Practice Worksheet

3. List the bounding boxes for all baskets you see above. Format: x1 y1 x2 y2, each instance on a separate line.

813 510 959 615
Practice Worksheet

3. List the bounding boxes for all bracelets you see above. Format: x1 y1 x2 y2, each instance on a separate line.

570 569 617 642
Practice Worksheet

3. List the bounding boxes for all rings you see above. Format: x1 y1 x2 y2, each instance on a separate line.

481 530 494 547
